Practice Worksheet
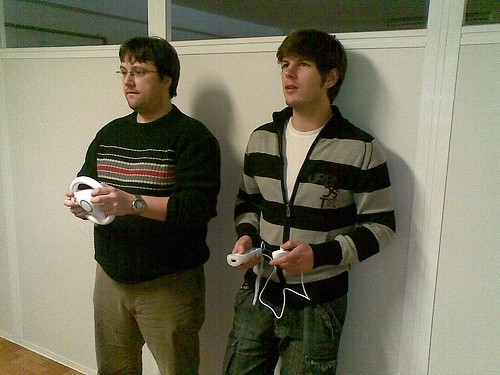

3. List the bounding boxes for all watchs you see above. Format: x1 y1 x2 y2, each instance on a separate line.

132 194 147 216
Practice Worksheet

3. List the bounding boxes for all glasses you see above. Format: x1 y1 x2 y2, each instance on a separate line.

115 65 161 77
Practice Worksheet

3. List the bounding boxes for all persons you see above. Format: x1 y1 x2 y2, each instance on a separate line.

222 27 397 374
63 34 221 374
